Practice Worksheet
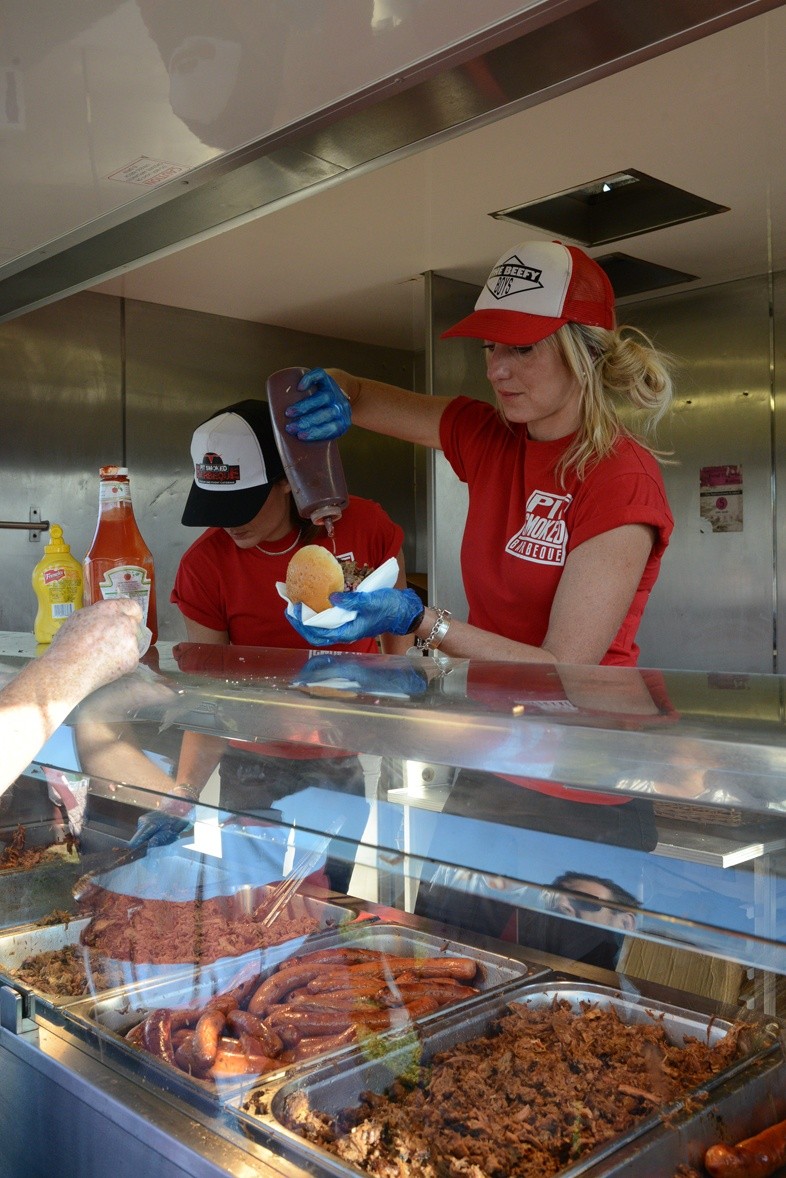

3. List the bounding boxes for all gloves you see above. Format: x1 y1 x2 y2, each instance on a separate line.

281 587 424 646
282 369 353 439
130 791 198 852
298 655 428 698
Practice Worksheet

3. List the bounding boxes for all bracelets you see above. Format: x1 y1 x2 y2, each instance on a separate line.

173 784 199 800
417 606 443 651
429 609 452 650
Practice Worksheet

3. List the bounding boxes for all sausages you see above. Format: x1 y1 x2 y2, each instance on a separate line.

125 945 486 1083
704 1119 786 1178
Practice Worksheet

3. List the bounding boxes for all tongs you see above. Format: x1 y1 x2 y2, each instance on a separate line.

253 817 344 927
73 841 147 904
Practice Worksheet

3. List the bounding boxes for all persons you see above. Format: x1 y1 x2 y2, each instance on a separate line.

126 399 412 898
282 239 678 984
0 597 142 798
413 665 674 981
546 871 640 933
71 671 197 857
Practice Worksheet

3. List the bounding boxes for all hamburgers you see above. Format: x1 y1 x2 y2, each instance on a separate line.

285 544 377 613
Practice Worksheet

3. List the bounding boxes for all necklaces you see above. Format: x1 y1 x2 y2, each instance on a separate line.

255 528 301 556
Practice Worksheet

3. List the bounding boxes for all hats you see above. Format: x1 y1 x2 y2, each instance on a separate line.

180 400 285 529
440 239 615 346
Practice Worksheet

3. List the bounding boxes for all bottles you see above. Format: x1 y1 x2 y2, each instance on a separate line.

31 525 82 643
82 468 158 646
266 366 348 536
